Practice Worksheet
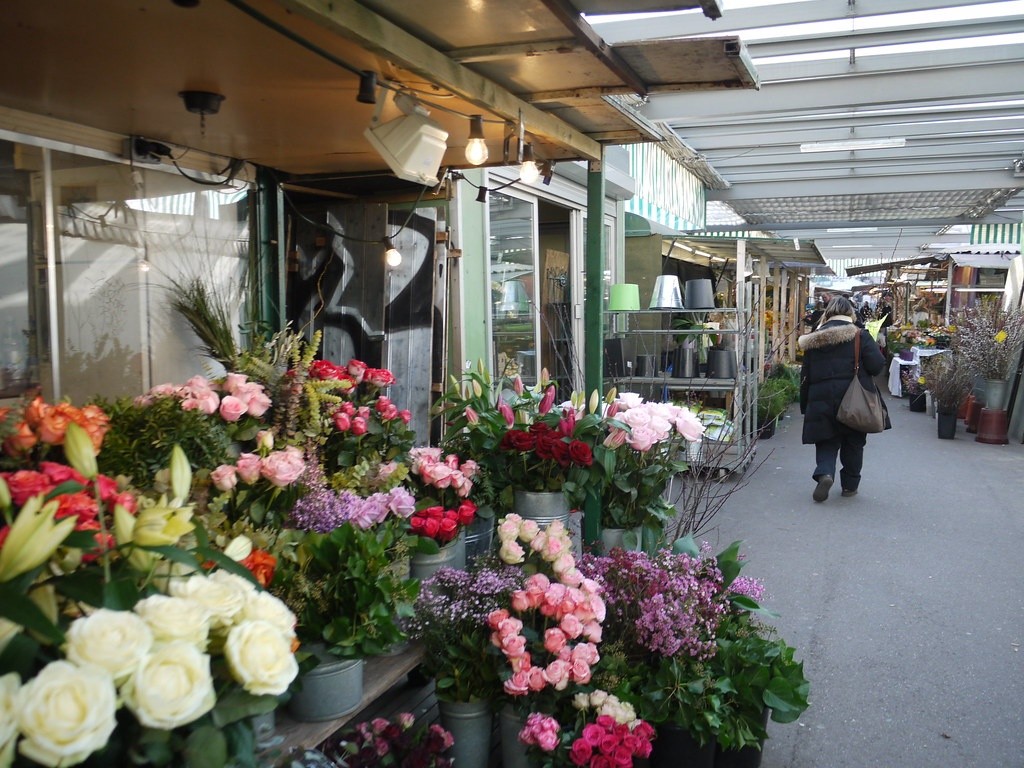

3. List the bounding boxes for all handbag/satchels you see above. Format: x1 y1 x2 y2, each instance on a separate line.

836 330 885 435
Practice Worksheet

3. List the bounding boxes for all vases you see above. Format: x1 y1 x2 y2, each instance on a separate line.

286 656 364 721
910 394 926 412
964 394 976 423
407 525 466 596
599 529 627 558
512 489 568 576
936 410 956 440
965 399 984 433
463 512 494 562
986 379 1012 413
956 402 967 417
438 698 493 768
975 407 1009 444
498 706 552 768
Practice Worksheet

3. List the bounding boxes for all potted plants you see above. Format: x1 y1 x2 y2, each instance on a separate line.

748 381 783 437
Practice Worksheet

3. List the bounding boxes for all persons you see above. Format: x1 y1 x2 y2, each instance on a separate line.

798 295 885 501
804 292 893 352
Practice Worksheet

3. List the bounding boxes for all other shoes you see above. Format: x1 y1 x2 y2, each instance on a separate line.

813 474 833 502
842 487 857 498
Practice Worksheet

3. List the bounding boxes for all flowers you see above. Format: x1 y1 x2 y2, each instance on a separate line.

0 266 808 768
955 296 1024 380
902 367 924 395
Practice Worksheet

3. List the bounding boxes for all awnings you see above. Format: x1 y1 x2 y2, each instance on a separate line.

949 254 1018 270
661 235 835 276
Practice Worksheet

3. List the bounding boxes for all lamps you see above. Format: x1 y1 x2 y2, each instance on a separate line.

357 69 377 103
361 87 451 187
369 555 410 658
